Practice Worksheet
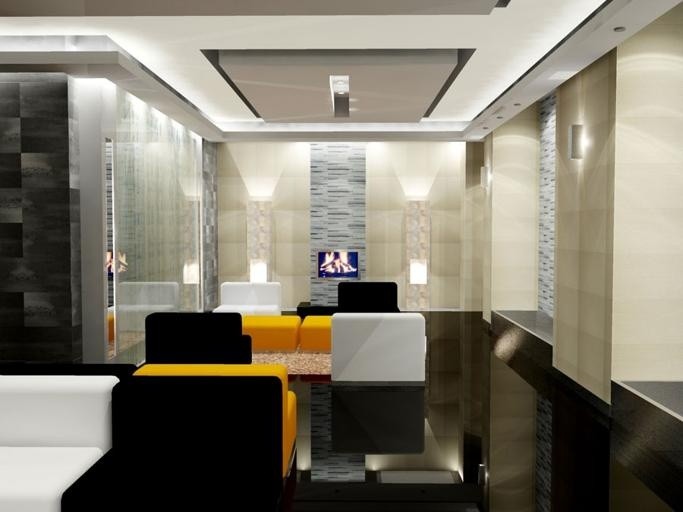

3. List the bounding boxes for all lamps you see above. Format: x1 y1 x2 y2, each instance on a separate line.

403 197 433 313
565 122 585 163
327 73 353 119
244 198 272 282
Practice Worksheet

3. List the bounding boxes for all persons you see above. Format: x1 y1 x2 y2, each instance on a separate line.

320 252 334 272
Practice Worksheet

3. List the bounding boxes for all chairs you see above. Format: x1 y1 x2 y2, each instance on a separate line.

0 368 123 512
104 280 179 330
135 355 300 472
212 278 285 315
140 309 252 368
332 279 403 312
4 357 134 384
60 372 289 511
328 310 425 390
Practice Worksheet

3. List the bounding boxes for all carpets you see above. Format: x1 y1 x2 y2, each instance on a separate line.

243 344 333 379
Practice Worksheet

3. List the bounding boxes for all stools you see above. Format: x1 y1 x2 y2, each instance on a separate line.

238 311 298 355
298 315 332 354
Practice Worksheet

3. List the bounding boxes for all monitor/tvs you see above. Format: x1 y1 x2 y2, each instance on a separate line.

316 249 359 280
